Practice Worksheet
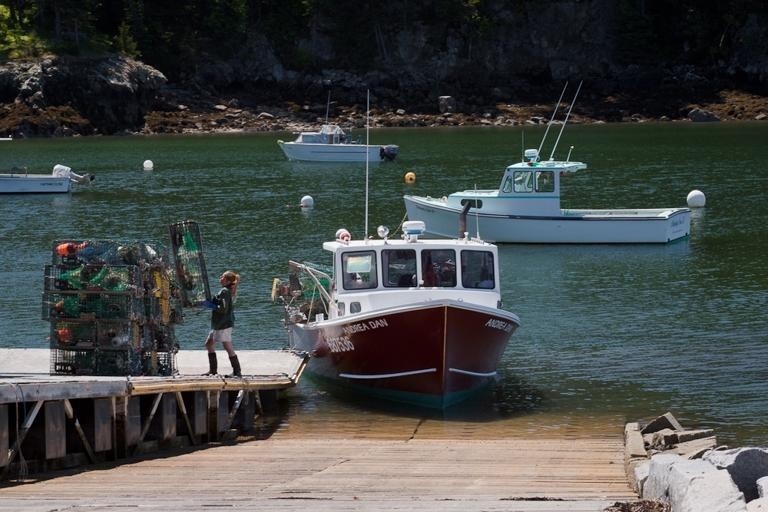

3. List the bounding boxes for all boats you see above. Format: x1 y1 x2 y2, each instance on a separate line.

404 160 691 242
1 166 93 194
277 126 398 166
281 221 521 411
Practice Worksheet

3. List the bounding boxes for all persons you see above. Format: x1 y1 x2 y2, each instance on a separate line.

397 260 439 288
478 272 494 289
196 269 241 378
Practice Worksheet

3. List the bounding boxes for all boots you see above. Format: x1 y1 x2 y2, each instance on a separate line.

224 354 241 378
201 352 217 377
